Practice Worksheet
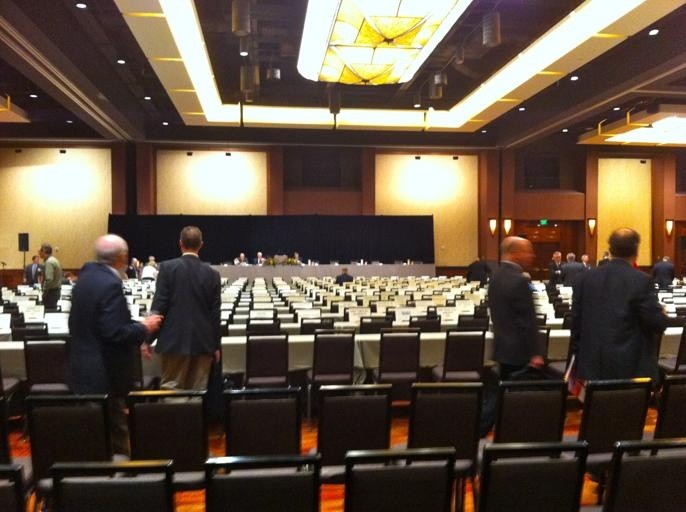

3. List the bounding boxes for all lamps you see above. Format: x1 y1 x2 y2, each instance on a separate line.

576 99 686 148
665 219 674 237
231 0 502 114
503 217 512 235
489 218 496 238
587 218 596 237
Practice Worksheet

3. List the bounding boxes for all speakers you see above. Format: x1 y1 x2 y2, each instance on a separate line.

18 232 29 252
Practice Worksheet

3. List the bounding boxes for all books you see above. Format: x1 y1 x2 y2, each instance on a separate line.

563 355 590 403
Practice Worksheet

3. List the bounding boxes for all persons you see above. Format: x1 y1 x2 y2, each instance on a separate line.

653 255 675 290
480 236 546 440
466 257 490 287
548 249 609 288
25 243 158 314
142 225 222 403
253 252 266 264
566 225 665 404
334 267 354 287
293 252 304 264
235 253 248 265
63 232 164 457
521 272 537 293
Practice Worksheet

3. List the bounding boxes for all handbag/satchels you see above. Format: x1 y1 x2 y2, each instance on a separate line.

516 366 563 388
